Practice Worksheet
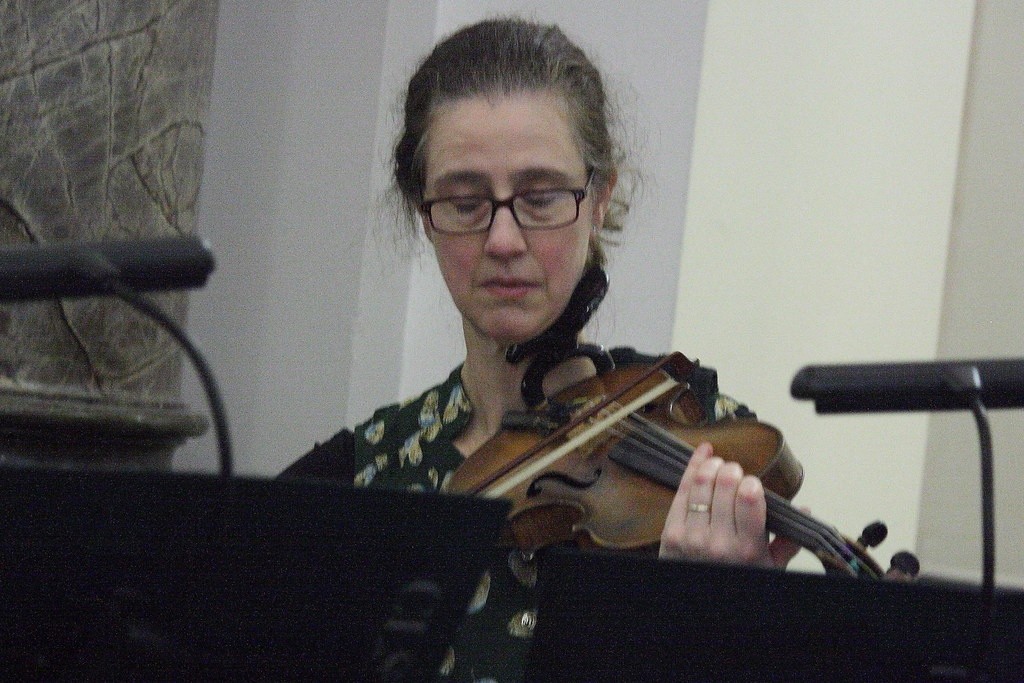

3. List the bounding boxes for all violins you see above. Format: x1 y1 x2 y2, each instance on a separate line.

441 357 920 583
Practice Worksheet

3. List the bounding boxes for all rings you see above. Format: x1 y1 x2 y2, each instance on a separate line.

687 503 711 513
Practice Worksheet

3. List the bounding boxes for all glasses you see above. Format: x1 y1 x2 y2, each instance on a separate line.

413 166 597 235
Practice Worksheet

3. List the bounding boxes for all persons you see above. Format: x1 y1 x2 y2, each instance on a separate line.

267 15 810 683
